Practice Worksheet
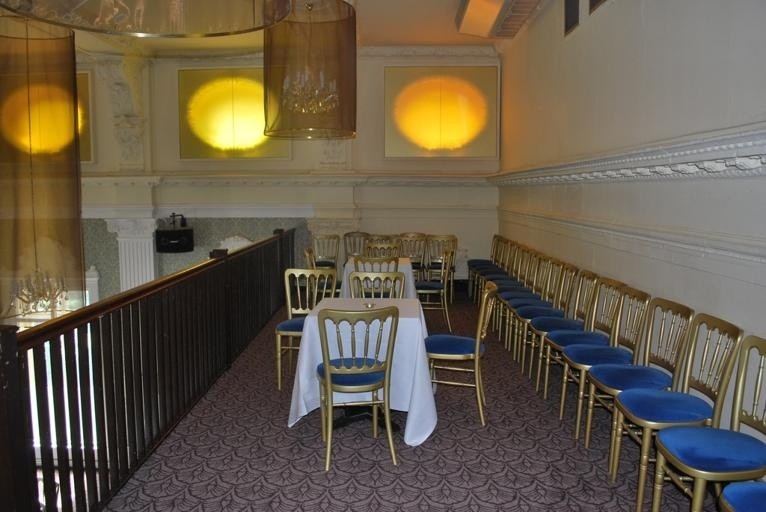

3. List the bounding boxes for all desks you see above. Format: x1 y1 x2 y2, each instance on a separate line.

286 297 438 447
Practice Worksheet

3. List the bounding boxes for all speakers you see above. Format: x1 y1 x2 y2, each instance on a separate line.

154 228 194 253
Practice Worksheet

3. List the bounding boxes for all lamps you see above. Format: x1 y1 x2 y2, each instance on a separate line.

262 0 359 142
1 0 86 328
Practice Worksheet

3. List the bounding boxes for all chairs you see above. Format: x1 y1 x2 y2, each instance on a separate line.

274 268 336 391
424 280 499 428
305 231 457 333
316 306 399 472
